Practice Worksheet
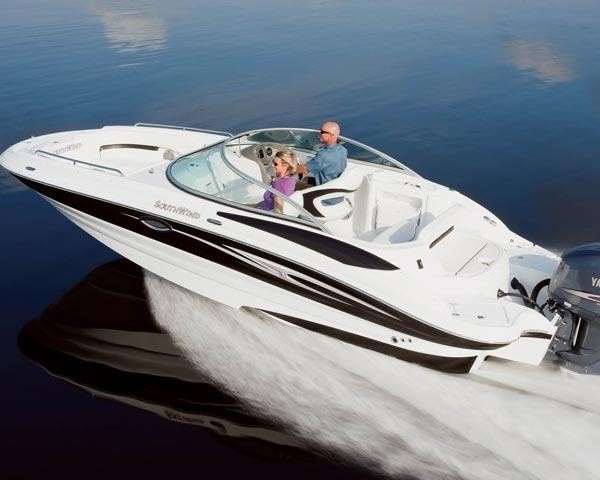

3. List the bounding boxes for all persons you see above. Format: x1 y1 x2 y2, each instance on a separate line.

247 149 299 215
297 121 348 186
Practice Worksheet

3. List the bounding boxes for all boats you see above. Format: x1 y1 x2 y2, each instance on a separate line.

17 258 323 454
0 127 600 379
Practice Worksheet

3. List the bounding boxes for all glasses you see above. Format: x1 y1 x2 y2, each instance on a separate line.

321 129 329 133
272 159 279 166
